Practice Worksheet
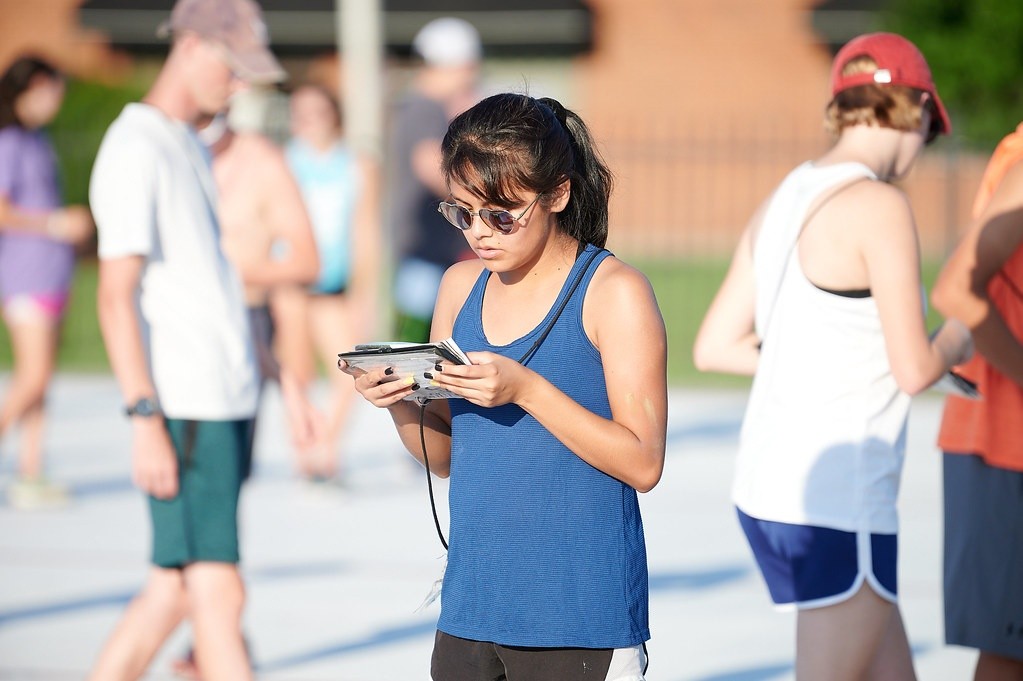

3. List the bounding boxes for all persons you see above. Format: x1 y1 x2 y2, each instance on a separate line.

0 55 95 512
84 0 286 680
930 120 1023 681
388 15 489 324
691 31 974 681
192 79 375 490
339 94 669 681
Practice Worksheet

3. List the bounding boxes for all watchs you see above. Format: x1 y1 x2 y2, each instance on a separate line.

125 398 161 416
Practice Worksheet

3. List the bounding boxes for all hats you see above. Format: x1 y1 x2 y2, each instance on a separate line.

156 0 287 83
832 33 954 136
413 17 482 64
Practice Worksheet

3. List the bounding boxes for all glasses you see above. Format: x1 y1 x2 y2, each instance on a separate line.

438 178 558 235
